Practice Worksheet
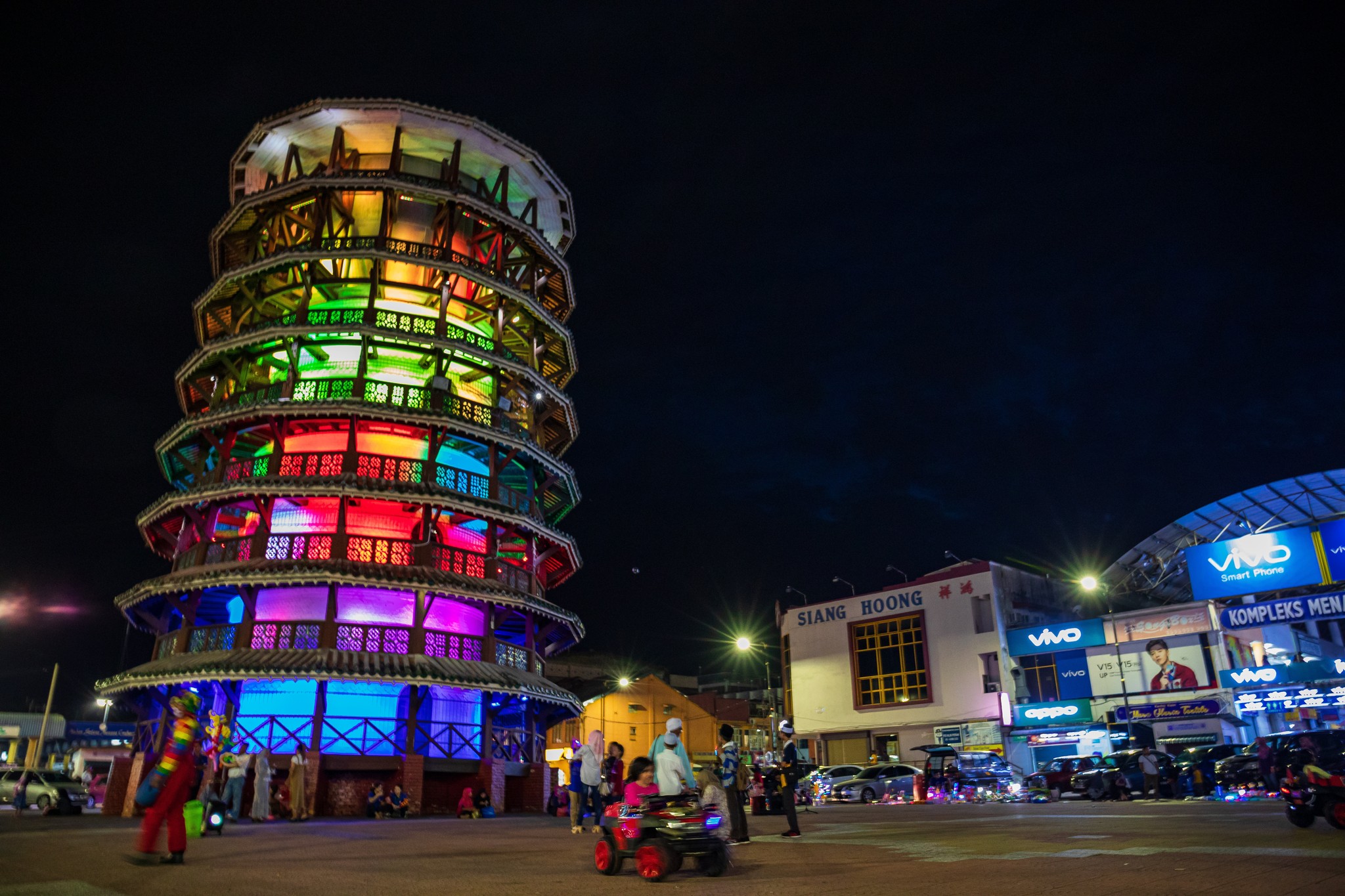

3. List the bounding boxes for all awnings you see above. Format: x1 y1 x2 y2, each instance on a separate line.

1010 722 1107 737
1156 732 1219 745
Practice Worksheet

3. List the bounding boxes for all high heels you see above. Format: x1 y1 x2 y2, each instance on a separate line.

572 826 583 835
591 825 601 833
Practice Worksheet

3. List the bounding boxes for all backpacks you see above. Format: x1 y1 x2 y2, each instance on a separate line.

724 746 750 790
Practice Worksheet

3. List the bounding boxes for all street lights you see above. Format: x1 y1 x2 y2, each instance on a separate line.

1077 574 1138 750
602 677 629 758
735 636 777 763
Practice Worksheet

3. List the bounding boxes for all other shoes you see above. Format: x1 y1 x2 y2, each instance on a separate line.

123 852 157 867
725 836 751 845
290 815 309 823
158 851 184 865
781 830 801 838
252 815 268 823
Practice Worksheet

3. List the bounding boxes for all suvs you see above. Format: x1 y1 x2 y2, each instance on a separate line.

592 791 730 883
1214 728 1345 789
0 766 90 816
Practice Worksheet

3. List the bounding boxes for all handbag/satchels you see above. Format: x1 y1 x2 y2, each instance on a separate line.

481 806 496 819
599 766 614 797
134 763 170 807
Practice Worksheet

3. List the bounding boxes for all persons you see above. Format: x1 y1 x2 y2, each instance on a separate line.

1138 745 1161 800
868 750 878 766
778 723 802 837
546 784 613 816
656 733 685 796
647 718 698 790
13 773 34 818
624 755 660 838
1087 761 1204 800
695 768 732 842
1145 639 1199 691
717 724 751 845
946 764 958 778
1255 737 1318 790
929 771 951 789
366 783 409 820
81 765 93 787
1074 762 1086 774
457 787 491 819
125 692 205 865
185 740 309 823
883 751 890 764
567 730 624 834
750 762 763 790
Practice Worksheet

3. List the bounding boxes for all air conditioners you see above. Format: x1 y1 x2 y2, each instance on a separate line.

987 682 1001 693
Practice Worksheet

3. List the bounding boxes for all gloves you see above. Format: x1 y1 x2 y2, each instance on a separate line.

148 773 162 789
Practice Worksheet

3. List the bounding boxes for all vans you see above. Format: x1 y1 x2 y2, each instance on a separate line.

909 743 1013 790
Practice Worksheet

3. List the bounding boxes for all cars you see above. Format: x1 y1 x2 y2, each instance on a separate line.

84 773 108 809
831 763 923 804
1070 748 1177 799
1022 754 1103 794
759 764 819 795
1166 742 1250 796
792 764 865 805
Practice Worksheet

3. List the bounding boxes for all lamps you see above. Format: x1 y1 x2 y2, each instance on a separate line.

885 565 908 582
1235 519 1251 534
786 585 808 605
430 375 451 390
832 575 854 595
497 396 513 413
943 551 962 563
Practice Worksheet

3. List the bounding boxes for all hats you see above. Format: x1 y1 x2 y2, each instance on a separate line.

663 732 678 746
170 691 203 714
666 716 682 732
